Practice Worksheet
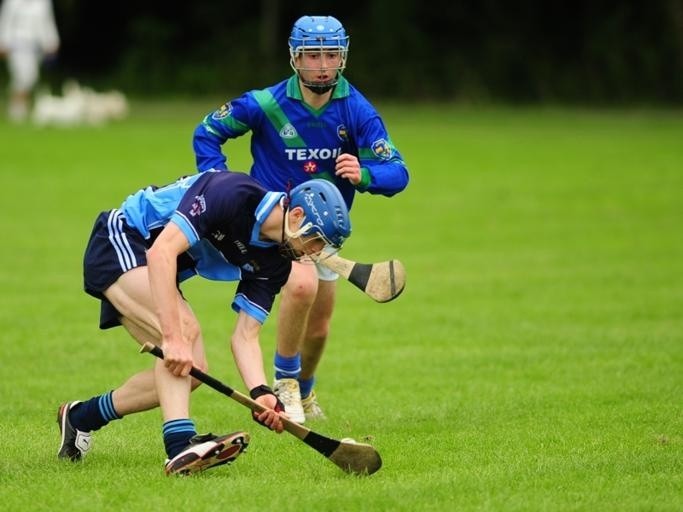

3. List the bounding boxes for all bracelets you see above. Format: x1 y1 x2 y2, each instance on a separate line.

249 384 273 399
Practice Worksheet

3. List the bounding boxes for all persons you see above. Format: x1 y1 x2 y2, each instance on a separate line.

59 170 352 476
0 0 61 122
193 13 408 421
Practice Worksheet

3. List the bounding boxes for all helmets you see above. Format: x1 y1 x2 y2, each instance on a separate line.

287 14 348 55
284 178 352 250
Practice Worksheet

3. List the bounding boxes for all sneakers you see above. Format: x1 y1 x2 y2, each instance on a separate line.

162 430 252 479
300 387 325 419
269 377 305 426
54 398 91 465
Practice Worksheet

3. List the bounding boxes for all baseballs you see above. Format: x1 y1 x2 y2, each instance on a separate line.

339 438 357 445
